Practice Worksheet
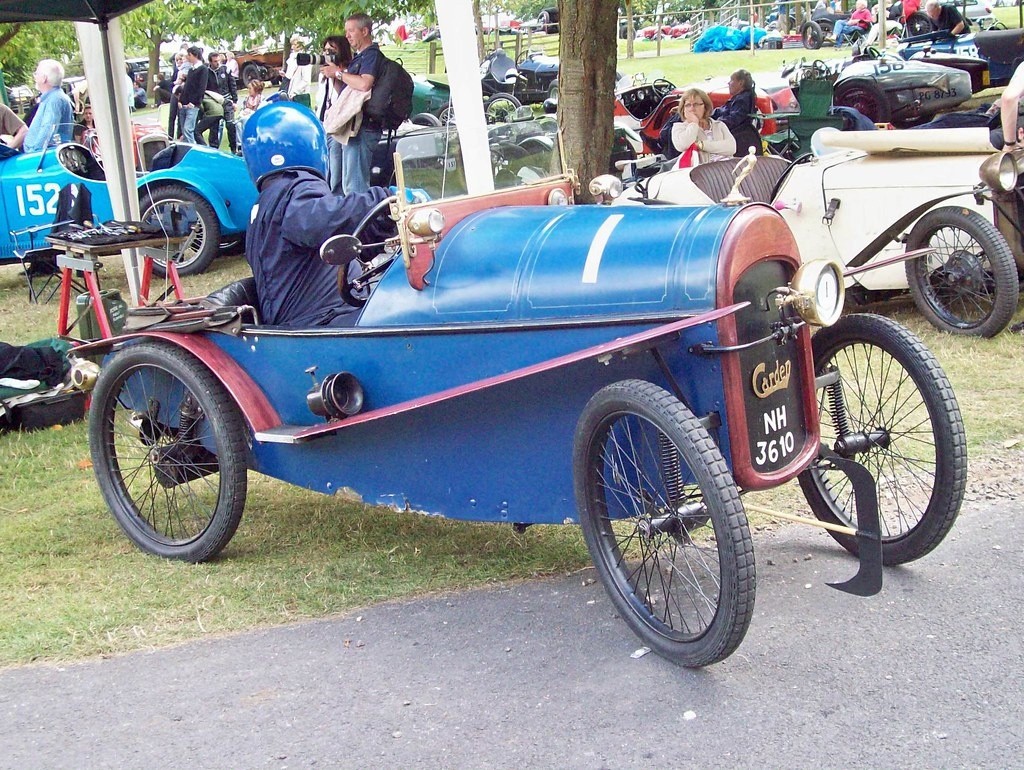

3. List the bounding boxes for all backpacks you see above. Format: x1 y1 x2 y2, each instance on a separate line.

356 49 415 130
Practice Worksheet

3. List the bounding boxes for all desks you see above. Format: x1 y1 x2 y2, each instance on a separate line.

45 227 189 341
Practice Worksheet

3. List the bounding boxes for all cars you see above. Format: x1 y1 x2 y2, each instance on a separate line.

355 0 1024 212
222 49 287 91
0 119 264 277
126 58 173 93
582 124 1024 339
48 115 968 672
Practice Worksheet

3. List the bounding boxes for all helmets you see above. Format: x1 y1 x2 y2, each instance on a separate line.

241 100 329 192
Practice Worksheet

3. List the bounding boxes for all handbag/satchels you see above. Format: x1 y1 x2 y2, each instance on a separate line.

813 60 840 83
798 71 834 118
278 76 290 94
0 342 63 388
671 143 700 169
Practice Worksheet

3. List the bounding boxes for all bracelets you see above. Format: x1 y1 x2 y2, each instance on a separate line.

1005 141 1017 145
338 71 343 81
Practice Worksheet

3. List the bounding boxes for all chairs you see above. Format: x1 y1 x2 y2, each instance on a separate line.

9 183 101 306
841 21 873 49
785 78 846 160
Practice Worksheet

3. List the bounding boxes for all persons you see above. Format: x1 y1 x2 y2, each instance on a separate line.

244 101 433 326
318 13 385 195
713 69 764 155
670 89 736 168
768 0 921 48
732 146 757 190
126 64 148 113
151 44 240 152
0 59 96 152
909 61 1024 151
237 35 313 141
926 0 970 33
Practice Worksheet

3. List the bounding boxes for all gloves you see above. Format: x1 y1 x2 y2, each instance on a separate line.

389 186 432 204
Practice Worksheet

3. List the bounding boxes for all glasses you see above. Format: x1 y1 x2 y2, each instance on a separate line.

323 47 334 53
684 102 705 108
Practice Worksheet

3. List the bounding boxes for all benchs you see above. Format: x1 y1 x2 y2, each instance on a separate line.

690 156 792 204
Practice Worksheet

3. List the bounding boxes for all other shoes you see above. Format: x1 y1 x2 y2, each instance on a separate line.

825 37 836 44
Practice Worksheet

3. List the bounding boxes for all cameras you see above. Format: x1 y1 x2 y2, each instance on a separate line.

298 52 342 66
